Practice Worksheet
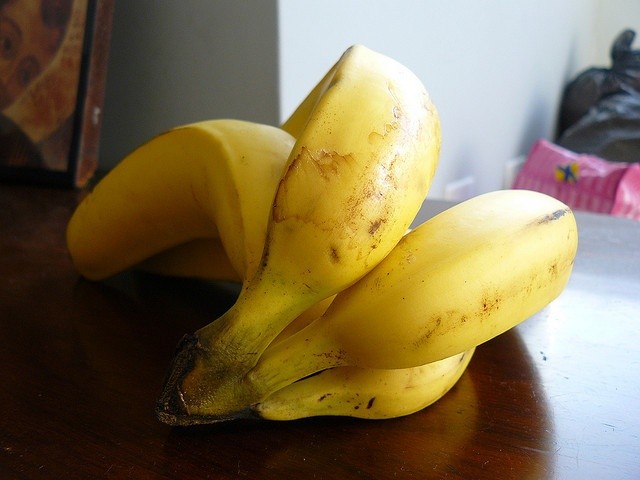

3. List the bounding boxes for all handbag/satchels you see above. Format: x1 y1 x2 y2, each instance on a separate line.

512 139 639 217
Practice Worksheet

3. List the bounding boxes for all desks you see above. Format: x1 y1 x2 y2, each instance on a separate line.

1 189 639 480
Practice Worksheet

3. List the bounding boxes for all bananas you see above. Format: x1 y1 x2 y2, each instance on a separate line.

63 42 580 430
